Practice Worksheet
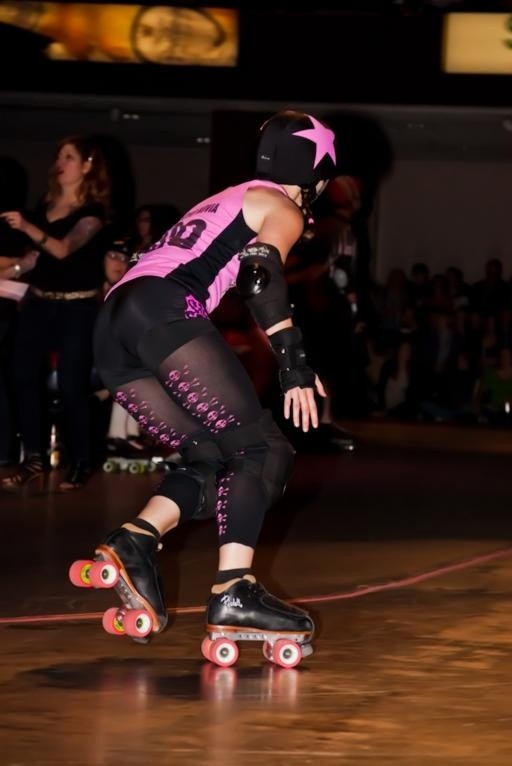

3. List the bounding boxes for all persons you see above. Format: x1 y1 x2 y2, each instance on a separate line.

209 177 510 459
2 203 179 471
0 128 111 492
88 106 347 637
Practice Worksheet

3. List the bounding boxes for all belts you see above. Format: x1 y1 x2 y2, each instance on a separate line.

28 285 99 301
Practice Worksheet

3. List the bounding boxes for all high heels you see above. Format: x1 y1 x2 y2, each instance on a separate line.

2 446 50 494
56 454 93 492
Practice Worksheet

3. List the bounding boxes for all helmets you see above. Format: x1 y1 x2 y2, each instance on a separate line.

255 110 345 191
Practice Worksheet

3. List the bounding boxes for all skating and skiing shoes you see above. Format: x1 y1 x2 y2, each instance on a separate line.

65 524 174 644
196 576 322 672
101 431 164 477
